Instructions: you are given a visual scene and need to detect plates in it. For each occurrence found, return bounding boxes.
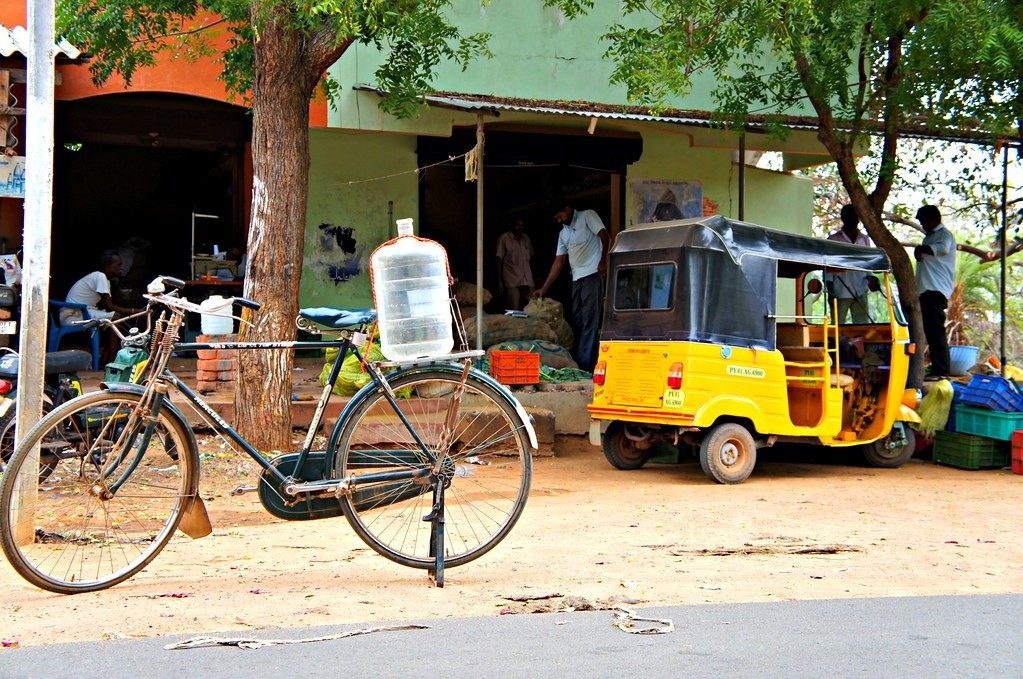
[197,253,209,257]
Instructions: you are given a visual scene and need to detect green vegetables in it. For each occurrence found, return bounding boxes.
[469,344,592,384]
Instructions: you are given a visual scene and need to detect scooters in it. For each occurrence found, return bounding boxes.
[0,287,191,485]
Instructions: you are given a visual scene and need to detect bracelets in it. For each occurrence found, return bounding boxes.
[132,308,134,314]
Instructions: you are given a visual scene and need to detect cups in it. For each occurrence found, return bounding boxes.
[214,245,219,255]
[218,253,223,261]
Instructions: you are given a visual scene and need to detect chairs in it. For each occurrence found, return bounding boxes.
[48,299,101,372]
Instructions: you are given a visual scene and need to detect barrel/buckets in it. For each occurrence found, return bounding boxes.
[201,295,233,335]
[948,345,978,376]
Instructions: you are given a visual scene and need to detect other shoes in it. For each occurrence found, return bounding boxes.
[98,359,122,374]
[925,371,949,380]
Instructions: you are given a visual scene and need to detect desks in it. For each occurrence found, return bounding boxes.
[164,279,243,342]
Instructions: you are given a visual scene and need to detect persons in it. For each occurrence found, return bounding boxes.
[914,205,956,382]
[824,203,870,324]
[533,199,610,373]
[496,219,535,310]
[59,249,150,367]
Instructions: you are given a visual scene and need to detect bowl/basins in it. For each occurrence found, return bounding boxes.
[200,275,206,281]
[211,276,218,282]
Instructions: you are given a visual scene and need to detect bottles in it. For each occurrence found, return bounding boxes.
[207,269,210,280]
[455,464,477,477]
[196,273,199,280]
[371,218,454,362]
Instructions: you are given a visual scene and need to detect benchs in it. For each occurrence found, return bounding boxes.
[775,321,854,387]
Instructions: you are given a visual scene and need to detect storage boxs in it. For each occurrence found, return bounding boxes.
[932,373,1023,475]
[489,349,540,384]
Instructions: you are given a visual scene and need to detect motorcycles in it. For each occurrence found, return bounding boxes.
[589,215,924,484]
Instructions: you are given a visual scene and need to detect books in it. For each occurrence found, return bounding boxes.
[512,314,527,317]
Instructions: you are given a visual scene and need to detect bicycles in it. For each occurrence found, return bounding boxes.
[0,276,539,594]
